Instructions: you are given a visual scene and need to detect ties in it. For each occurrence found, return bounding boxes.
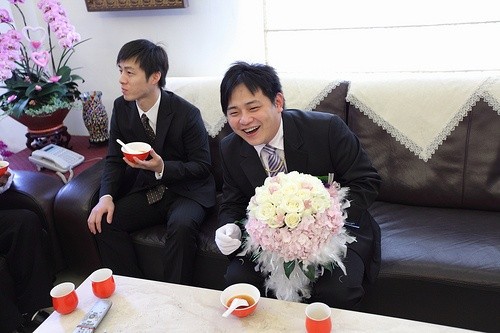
[260,144,287,177]
[139,114,166,205]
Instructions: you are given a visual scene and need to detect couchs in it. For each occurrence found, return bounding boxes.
[0,171,64,271]
[54,76,500,333]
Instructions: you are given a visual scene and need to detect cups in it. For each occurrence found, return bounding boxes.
[49,281,78,314]
[91,268,115,297]
[305,301,332,333]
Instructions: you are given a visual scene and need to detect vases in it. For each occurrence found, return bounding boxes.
[3,108,73,134]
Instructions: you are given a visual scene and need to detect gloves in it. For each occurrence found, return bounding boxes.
[216,223,242,255]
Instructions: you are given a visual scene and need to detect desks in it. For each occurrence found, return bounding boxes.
[32,269,483,333]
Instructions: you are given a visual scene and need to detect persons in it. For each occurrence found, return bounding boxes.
[0,156,51,333]
[216,61,384,312]
[86,39,216,286]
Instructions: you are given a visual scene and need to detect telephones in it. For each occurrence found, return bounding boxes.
[28,143,85,173]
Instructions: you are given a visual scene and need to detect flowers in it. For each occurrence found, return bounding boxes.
[243,171,350,279]
[0,0,92,118]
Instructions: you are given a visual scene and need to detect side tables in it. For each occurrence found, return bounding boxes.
[3,135,109,179]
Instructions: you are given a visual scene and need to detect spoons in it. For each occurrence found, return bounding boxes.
[222,297,248,317]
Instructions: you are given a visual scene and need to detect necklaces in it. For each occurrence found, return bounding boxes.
[262,150,286,173]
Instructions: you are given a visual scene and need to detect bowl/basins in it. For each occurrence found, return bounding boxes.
[0,161,9,176]
[220,283,260,317]
[121,141,151,162]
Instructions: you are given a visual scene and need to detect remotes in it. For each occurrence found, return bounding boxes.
[73,299,113,333]
